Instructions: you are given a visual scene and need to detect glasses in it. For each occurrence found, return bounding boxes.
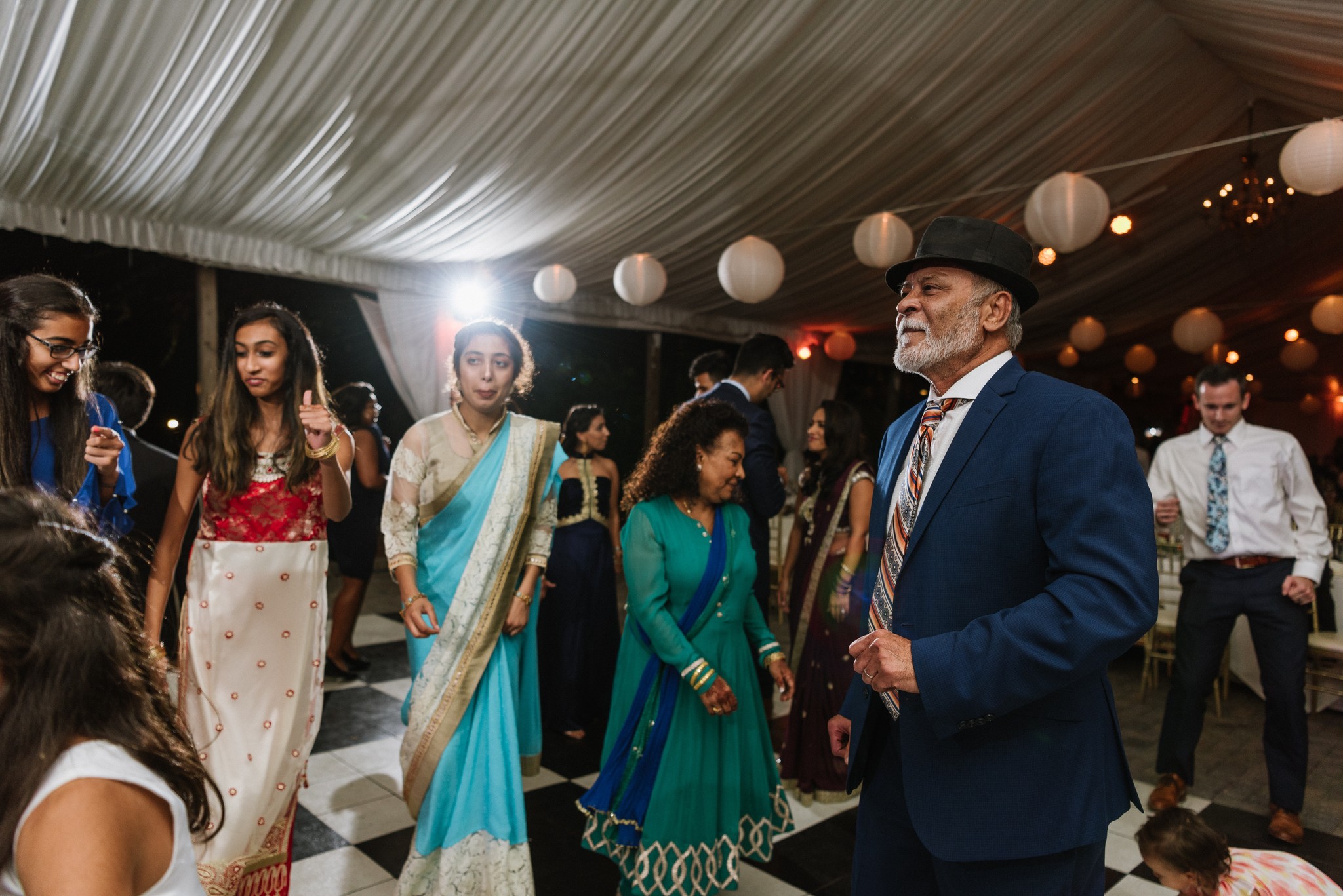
[24,331,101,361]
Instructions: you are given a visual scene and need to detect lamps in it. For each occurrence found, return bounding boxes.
[853,212,913,270]
[532,263,578,306]
[825,332,856,362]
[717,234,786,305]
[612,254,668,307]
[1025,114,1343,431]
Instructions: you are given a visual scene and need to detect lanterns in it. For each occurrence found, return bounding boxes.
[826,333,856,361]
[1056,286,1343,428]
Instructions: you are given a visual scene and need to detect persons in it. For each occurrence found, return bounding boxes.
[0,479,228,896]
[776,399,876,811]
[1133,806,1342,896]
[826,210,1162,896]
[379,319,568,896]
[323,380,392,681]
[150,304,355,896]
[573,394,795,896]
[1143,362,1332,844]
[687,329,796,748]
[537,403,626,751]
[87,359,202,670]
[0,272,138,548]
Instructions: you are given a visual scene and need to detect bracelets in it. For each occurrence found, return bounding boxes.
[514,591,533,606]
[145,642,168,667]
[304,432,342,462]
[757,641,785,668]
[399,592,427,620]
[680,658,715,691]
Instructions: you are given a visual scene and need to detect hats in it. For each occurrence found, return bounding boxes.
[882,211,1044,316]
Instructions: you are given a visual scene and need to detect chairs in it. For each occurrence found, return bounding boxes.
[1136,516,1343,729]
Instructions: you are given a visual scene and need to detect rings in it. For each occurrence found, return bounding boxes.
[864,667,874,679]
[713,707,722,714]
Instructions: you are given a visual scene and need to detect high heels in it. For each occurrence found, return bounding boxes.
[323,653,359,682]
[340,649,369,671]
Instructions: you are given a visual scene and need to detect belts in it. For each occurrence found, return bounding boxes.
[1213,549,1280,573]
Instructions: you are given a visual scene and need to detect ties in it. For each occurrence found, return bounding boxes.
[1204,433,1239,553]
[862,398,971,719]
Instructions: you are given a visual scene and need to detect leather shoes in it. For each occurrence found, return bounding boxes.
[1267,807,1305,846]
[1148,774,1187,814]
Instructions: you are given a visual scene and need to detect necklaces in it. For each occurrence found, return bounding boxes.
[681,496,715,523]
